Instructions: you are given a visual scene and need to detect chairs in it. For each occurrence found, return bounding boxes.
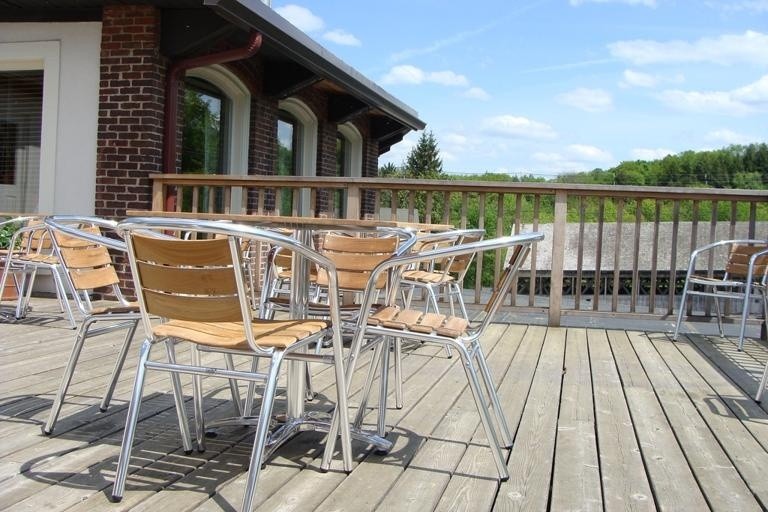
[673,239,767,353]
